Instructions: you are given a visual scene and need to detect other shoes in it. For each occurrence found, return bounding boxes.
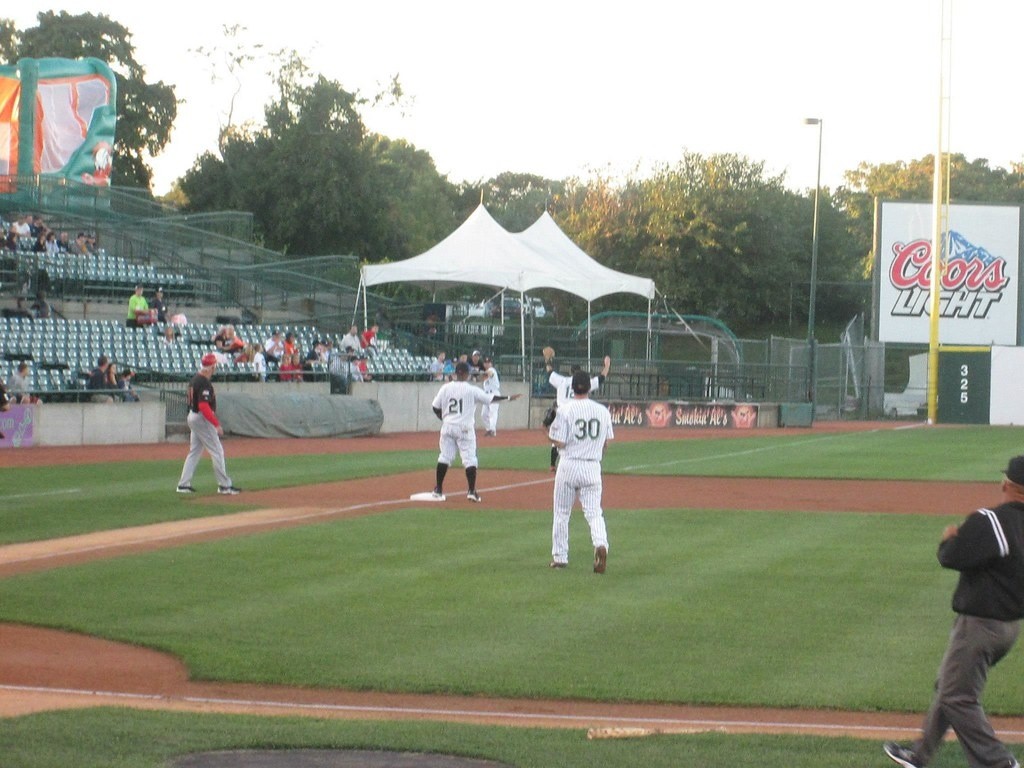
[484,430,494,437]
[593,546,606,574]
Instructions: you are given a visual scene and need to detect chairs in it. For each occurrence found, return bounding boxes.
[0,229,456,400]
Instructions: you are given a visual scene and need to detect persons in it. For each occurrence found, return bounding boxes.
[0,362,43,440]
[433,350,485,382]
[87,356,139,402]
[542,346,609,471]
[481,357,499,436]
[177,354,242,496]
[126,285,186,341]
[881,456,1024,768]
[213,324,379,383]
[433,363,522,503]
[547,371,614,574]
[0,213,101,256]
[543,401,557,473]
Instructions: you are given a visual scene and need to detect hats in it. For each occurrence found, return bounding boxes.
[472,350,480,357]
[351,356,359,361]
[202,354,218,366]
[483,357,492,363]
[1001,456,1024,485]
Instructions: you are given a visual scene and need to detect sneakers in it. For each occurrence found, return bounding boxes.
[176,485,195,492]
[432,491,442,498]
[883,741,923,768]
[549,562,567,567]
[1009,755,1020,768]
[217,486,240,495]
[467,490,481,502]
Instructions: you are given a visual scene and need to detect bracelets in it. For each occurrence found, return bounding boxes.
[508,395,510,401]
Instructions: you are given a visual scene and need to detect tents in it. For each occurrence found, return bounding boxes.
[361,189,591,376]
[506,206,658,376]
